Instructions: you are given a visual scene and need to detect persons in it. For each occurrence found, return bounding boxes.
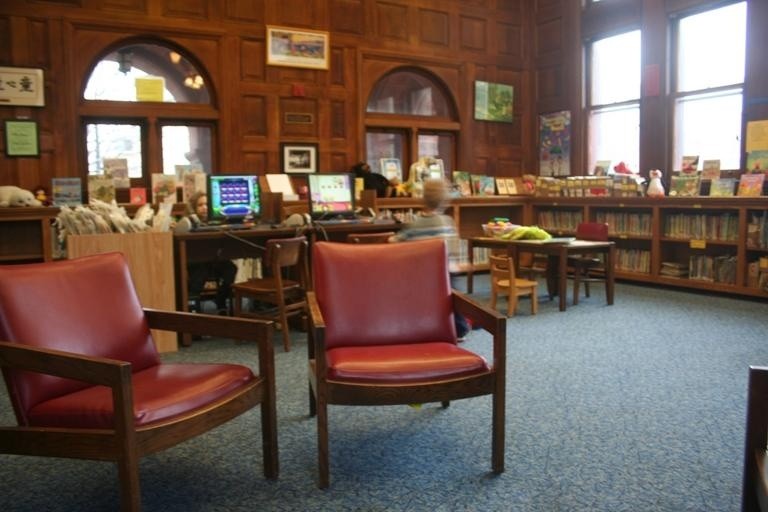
[187,191,236,341]
[387,179,470,342]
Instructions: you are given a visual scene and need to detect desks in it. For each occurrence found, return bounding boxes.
[466,237,621,311]
[172,218,409,348]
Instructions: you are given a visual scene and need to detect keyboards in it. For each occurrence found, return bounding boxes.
[191,225,250,232]
[315,220,360,226]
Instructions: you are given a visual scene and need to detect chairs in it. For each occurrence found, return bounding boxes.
[346,232,395,244]
[485,253,540,319]
[192,271,232,315]
[233,237,312,352]
[0,251,280,512]
[307,237,507,490]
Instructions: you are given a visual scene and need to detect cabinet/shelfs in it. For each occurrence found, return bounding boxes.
[376,197,767,301]
[0,207,60,262]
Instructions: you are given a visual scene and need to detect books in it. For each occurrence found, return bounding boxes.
[452,156,768,284]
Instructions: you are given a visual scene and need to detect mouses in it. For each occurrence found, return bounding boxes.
[396,218,403,223]
[271,222,279,228]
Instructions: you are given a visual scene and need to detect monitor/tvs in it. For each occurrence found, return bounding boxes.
[307,173,355,216]
[207,174,262,220]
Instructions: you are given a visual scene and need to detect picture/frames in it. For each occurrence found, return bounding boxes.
[280,142,319,174]
[266,24,330,70]
[380,158,402,183]
[536,108,572,177]
[5,119,40,155]
[0,67,44,107]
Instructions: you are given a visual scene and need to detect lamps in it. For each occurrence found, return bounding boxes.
[116,47,134,76]
